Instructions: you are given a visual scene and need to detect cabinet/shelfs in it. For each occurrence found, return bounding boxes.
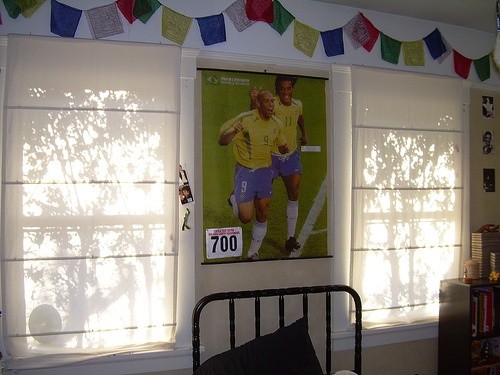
[437,277,500,375]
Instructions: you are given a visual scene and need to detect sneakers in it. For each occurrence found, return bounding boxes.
[284,236,301,250]
[228,190,235,208]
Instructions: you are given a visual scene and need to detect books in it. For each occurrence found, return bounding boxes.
[471,287,500,337]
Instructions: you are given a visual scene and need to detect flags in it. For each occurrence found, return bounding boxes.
[3,0,500,81]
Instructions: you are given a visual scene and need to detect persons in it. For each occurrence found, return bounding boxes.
[248,73,307,250]
[483,131,493,154]
[218,90,291,260]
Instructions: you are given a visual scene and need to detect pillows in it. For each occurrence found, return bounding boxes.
[196,317,324,375]
